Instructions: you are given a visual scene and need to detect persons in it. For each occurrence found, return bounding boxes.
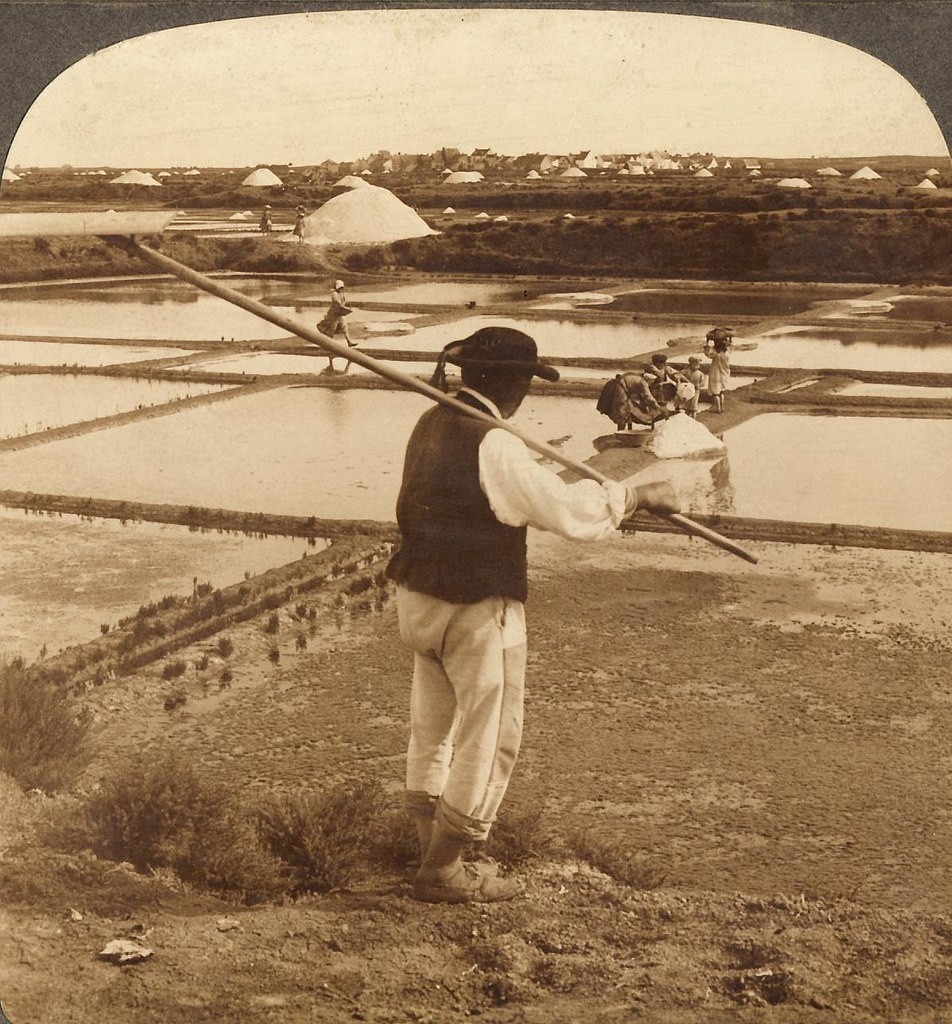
[597,332,734,433]
[322,356,353,376]
[259,205,272,237]
[317,278,359,346]
[293,204,306,242]
[388,326,682,902]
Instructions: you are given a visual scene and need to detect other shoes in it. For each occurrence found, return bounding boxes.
[411,857,526,903]
[348,343,358,347]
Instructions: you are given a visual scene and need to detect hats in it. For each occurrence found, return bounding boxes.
[651,354,668,363]
[688,356,703,362]
[333,279,344,290]
[429,327,560,393]
